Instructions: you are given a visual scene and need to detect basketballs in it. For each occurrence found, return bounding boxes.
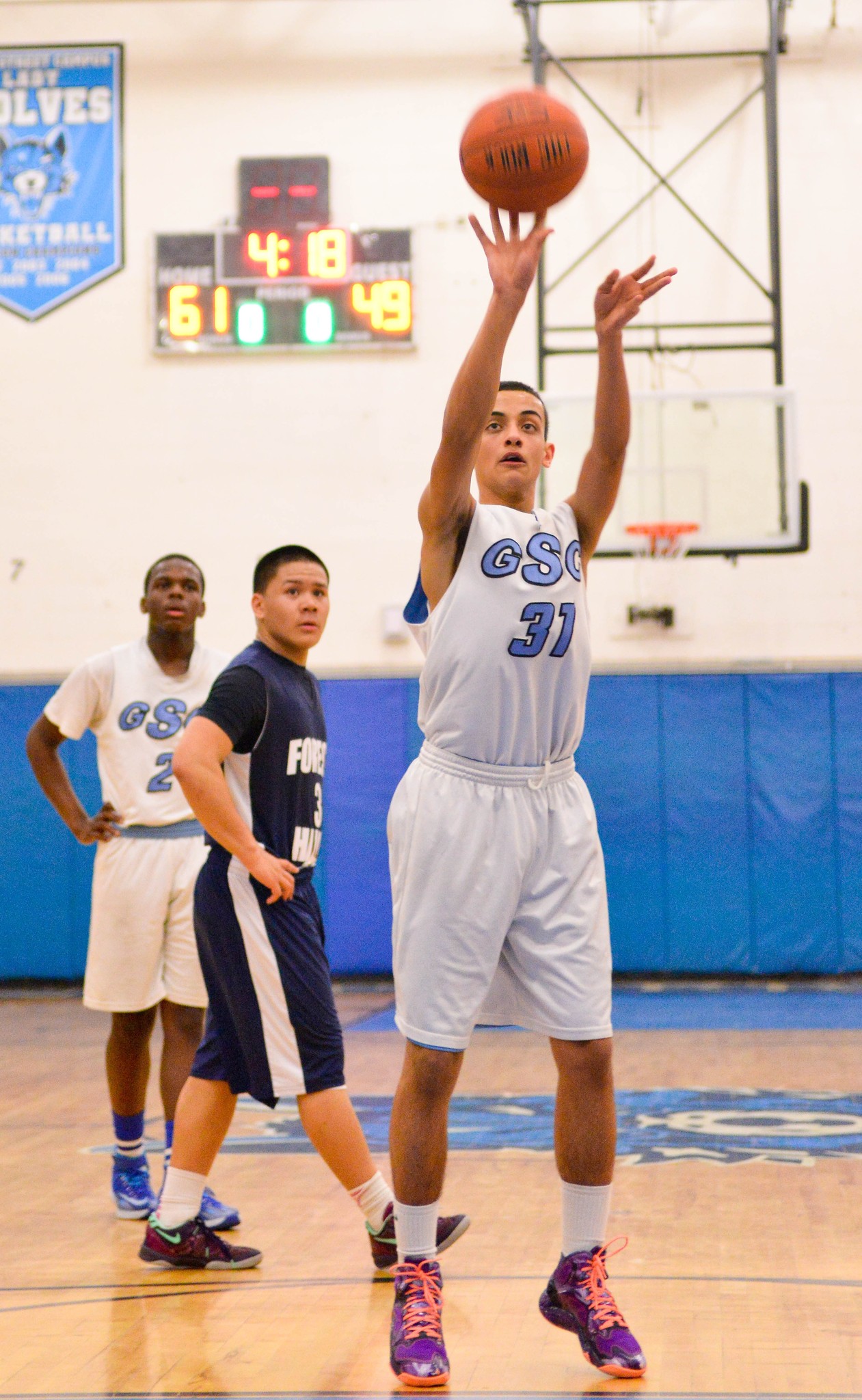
[459,89,591,213]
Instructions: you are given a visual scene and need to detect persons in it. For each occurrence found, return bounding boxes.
[141,545,472,1281]
[24,549,247,1233]
[386,196,683,1396]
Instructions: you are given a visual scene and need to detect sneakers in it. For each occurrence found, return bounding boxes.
[163,1175,239,1232]
[388,1256,451,1386]
[111,1162,159,1219]
[366,1200,471,1269]
[537,1237,646,1377]
[137,1213,261,1270]
